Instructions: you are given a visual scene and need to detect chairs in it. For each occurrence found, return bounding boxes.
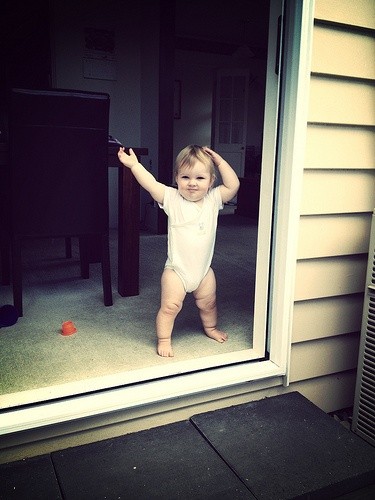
[2,83,114,319]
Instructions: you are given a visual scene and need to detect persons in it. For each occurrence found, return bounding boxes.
[119,144,242,357]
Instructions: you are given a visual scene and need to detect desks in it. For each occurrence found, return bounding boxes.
[105,134,149,300]
